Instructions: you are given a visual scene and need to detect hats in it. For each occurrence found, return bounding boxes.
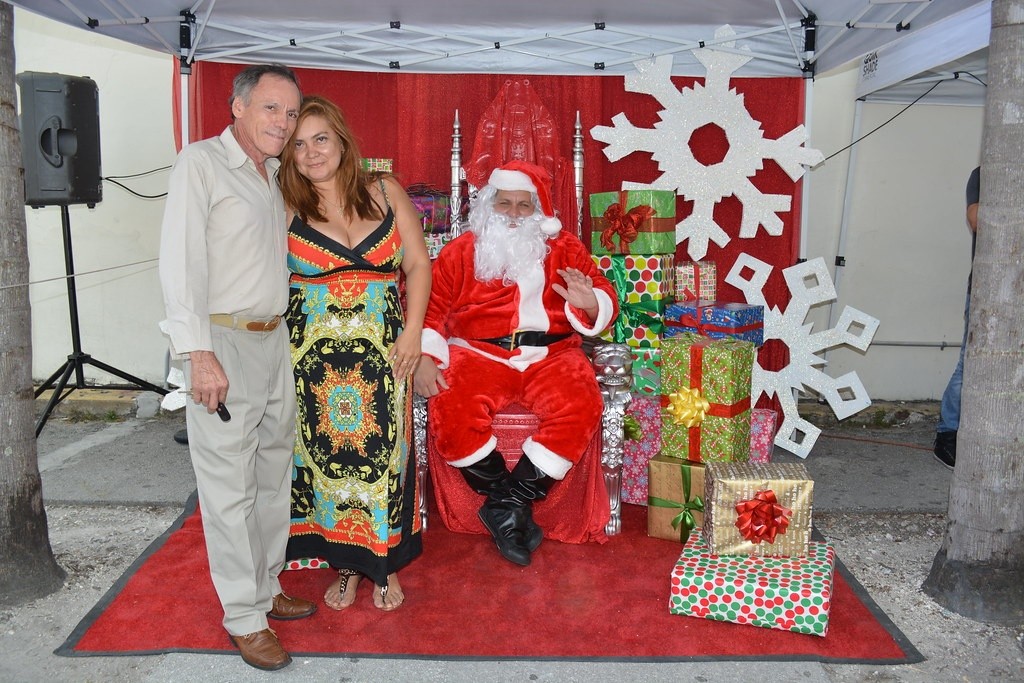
[487,161,561,236]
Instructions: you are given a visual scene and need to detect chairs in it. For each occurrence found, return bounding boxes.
[414,109,632,535]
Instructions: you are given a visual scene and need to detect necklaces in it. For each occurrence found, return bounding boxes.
[311,186,344,218]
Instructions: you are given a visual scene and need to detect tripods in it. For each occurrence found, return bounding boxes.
[34,203,171,442]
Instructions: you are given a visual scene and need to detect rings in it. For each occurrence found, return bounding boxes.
[418,392,424,395]
[402,360,408,365]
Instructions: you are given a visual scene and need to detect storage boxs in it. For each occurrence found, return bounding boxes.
[405,183,469,233]
[668,527,836,637]
[589,189,814,558]
[423,233,453,260]
[357,157,393,174]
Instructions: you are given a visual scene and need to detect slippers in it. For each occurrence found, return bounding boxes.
[372,572,400,611]
[324,571,359,609]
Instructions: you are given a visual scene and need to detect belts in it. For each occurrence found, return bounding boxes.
[476,329,572,353]
[209,312,282,331]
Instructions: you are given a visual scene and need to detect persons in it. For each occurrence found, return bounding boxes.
[932,165,981,463]
[157,63,317,670]
[276,96,432,610]
[414,160,619,568]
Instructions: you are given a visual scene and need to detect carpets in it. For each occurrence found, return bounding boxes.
[53,489,929,665]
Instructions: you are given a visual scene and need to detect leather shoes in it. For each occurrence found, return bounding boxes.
[266,593,317,621]
[229,629,292,671]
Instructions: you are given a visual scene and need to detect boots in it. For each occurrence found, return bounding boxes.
[476,451,554,566]
[459,447,544,553]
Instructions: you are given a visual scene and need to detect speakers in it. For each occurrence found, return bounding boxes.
[16,71,102,208]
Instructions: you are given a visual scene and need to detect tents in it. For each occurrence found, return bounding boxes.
[5,0,991,406]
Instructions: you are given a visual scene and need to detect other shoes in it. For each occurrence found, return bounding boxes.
[932,435,957,468]
